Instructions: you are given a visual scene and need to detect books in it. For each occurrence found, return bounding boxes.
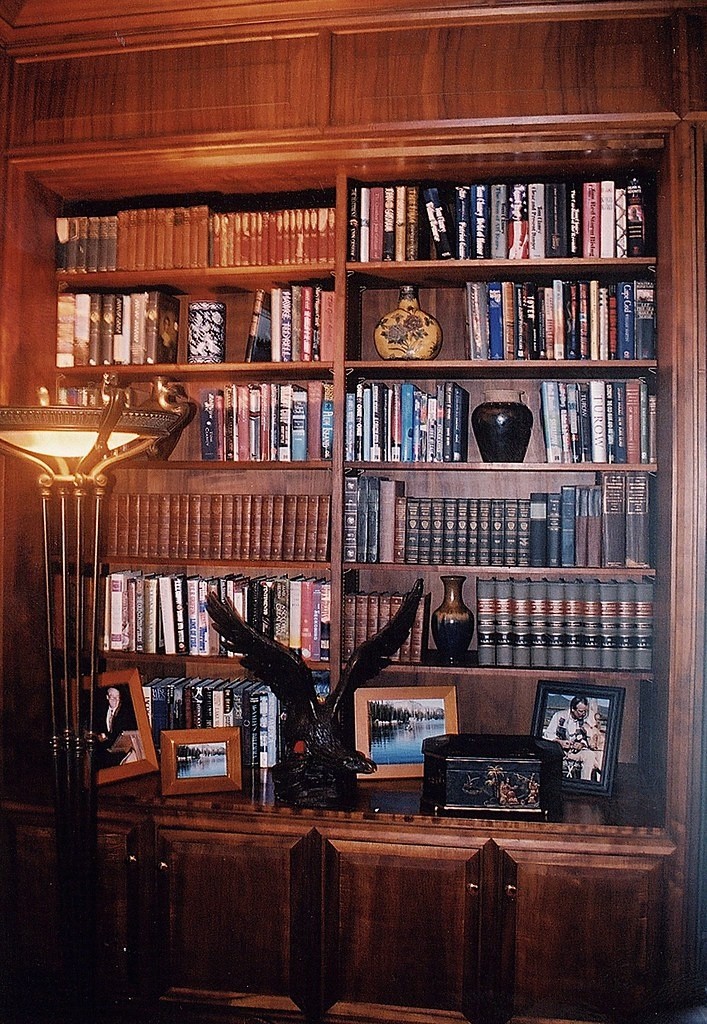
[199,380,337,464]
[343,470,651,569]
[102,491,334,562]
[343,377,469,462]
[347,176,656,261]
[476,576,653,671]
[53,206,339,274]
[137,678,325,805]
[539,378,658,465]
[107,572,330,660]
[465,276,658,362]
[344,592,431,665]
[55,286,333,366]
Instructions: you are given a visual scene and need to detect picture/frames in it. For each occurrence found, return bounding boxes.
[158,724,243,797]
[532,680,627,798]
[59,667,160,787]
[420,731,563,822]
[353,686,457,780]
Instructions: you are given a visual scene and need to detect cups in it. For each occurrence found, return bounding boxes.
[187,301,225,364]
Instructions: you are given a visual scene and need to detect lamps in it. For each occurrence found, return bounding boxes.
[0,373,199,1024]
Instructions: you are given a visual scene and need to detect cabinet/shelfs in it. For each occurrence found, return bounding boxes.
[0,106,707,1023]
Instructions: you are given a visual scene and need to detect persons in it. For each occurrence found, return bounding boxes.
[546,695,604,778]
[94,689,128,768]
